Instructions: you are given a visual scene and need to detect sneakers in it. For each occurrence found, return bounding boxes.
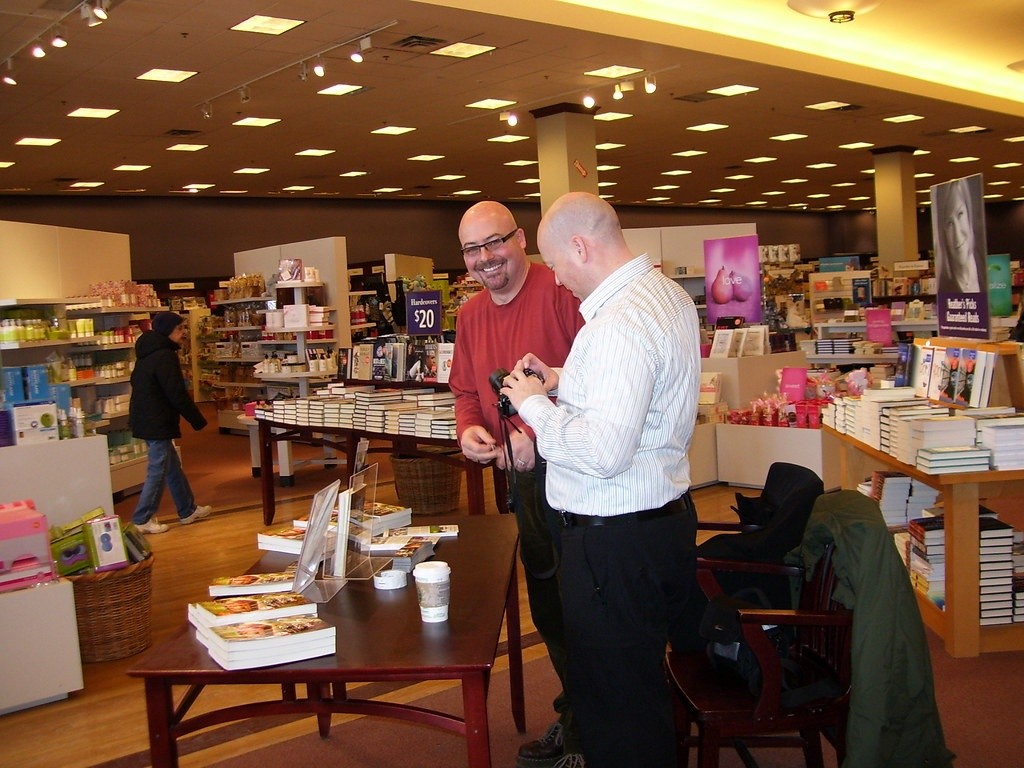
[133,520,168,534]
[179,505,212,523]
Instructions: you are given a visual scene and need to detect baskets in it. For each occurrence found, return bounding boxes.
[58,552,156,663]
[390,452,462,515]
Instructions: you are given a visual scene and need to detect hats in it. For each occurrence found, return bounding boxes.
[152,312,184,336]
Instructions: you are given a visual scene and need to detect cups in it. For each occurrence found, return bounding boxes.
[412,561,451,622]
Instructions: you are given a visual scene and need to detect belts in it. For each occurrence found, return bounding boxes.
[549,492,693,527]
[526,464,546,476]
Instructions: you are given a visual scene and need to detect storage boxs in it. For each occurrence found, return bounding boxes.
[48,506,152,577]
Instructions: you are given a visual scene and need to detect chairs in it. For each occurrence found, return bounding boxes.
[665,520,854,768]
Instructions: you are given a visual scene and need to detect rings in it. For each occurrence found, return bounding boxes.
[518,459,525,466]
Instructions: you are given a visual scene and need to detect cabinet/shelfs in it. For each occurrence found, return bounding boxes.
[348,254,486,341]
[208,282,342,434]
[660,261,1024,659]
[0,298,181,496]
[177,308,212,401]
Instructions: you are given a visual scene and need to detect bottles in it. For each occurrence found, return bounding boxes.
[71,329,130,378]
[57,407,86,440]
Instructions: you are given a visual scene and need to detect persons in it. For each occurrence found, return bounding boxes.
[936,178,986,294]
[447,201,586,768]
[500,192,702,768]
[125,312,212,532]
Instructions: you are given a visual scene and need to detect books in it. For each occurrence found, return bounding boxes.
[253,382,457,439]
[823,386,1024,475]
[187,572,336,672]
[800,337,863,354]
[857,471,1024,626]
[881,346,898,354]
[337,342,455,383]
[257,501,413,555]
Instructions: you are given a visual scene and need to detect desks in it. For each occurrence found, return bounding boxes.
[238,411,509,525]
[125,516,526,768]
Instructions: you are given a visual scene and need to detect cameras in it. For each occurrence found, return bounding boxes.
[489,368,544,418]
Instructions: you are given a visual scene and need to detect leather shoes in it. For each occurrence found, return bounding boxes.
[515,721,573,768]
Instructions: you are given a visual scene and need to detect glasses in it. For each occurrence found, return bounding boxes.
[460,229,518,258]
